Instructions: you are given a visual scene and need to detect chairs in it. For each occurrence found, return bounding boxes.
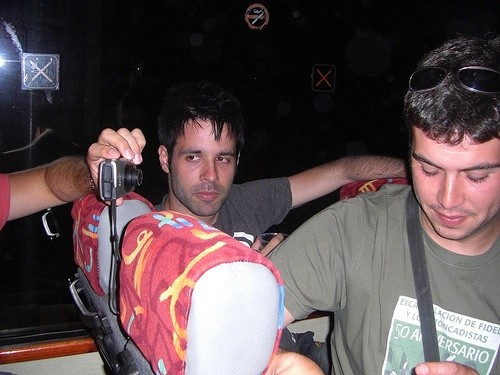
[70,191,285,375]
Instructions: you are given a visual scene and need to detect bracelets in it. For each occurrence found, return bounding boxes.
[88,175,95,192]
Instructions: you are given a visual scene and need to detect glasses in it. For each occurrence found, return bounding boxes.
[408,66,500,96]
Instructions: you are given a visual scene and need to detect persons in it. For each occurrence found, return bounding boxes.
[0,128,145,228]
[154,87,406,375]
[264,37,500,375]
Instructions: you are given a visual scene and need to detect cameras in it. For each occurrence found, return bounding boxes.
[99,157,143,202]
